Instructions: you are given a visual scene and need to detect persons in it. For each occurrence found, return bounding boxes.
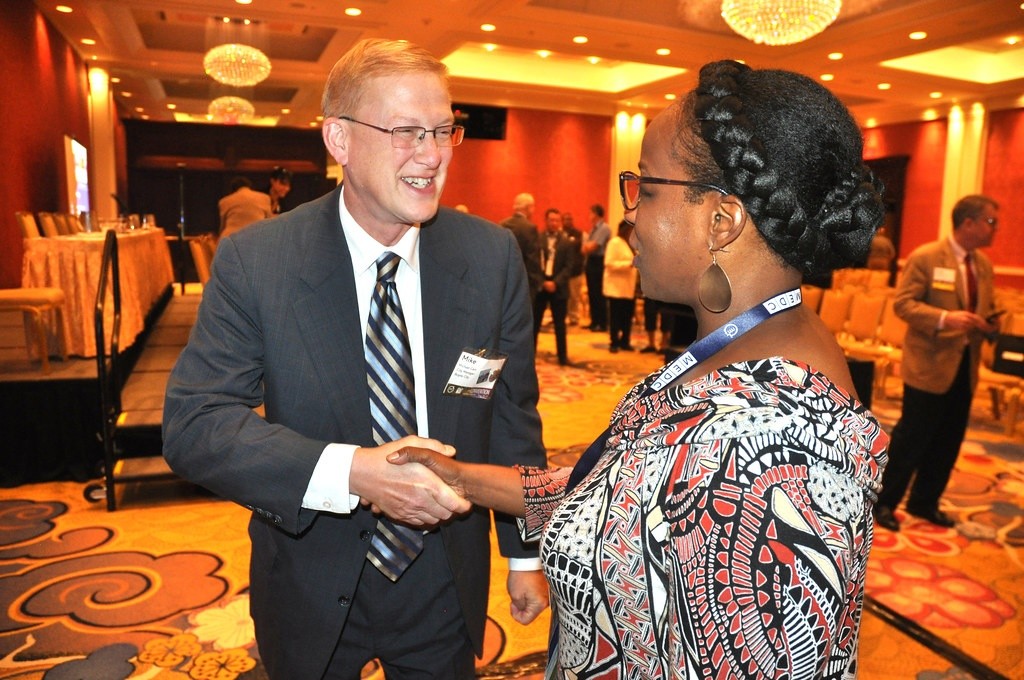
[531,208,577,364]
[219,178,271,236]
[876,195,1005,529]
[847,236,896,407]
[563,212,589,326]
[161,38,551,680]
[603,219,640,352]
[581,204,612,332]
[359,59,893,679]
[268,166,293,215]
[642,297,674,354]
[501,193,542,304]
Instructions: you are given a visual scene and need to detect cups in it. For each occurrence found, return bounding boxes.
[128,214,140,231]
[142,214,156,230]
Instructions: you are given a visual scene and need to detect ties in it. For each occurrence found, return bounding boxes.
[589,225,597,239]
[964,254,978,342]
[366,251,424,582]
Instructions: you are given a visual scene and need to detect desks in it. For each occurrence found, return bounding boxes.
[19,226,175,360]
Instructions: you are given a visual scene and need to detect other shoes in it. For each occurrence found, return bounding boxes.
[582,322,608,332]
[640,345,657,354]
[876,510,900,532]
[927,510,956,528]
[621,346,635,351]
[609,346,618,353]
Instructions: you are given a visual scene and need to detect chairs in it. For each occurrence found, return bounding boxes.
[794,267,1024,429]
[0,203,218,374]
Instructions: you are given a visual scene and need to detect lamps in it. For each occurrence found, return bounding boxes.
[207,96,256,124]
[721,0,842,48]
[204,43,272,86]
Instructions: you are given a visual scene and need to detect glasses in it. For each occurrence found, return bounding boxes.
[339,116,465,149]
[978,215,998,227]
[619,170,729,211]
